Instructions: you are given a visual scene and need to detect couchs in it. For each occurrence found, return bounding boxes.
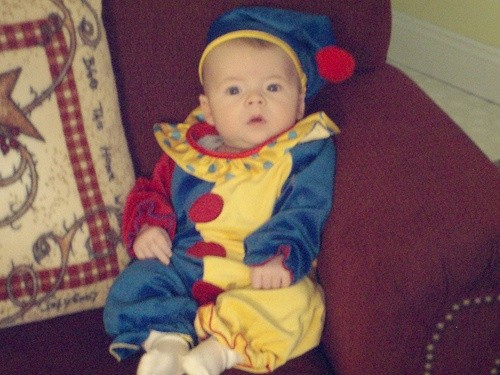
[1,1,500,375]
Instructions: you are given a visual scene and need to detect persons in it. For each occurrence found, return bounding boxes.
[102,5,343,375]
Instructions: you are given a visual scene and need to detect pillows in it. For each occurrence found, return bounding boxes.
[0,0,137,330]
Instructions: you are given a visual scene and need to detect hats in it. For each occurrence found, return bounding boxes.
[198,9,356,97]
